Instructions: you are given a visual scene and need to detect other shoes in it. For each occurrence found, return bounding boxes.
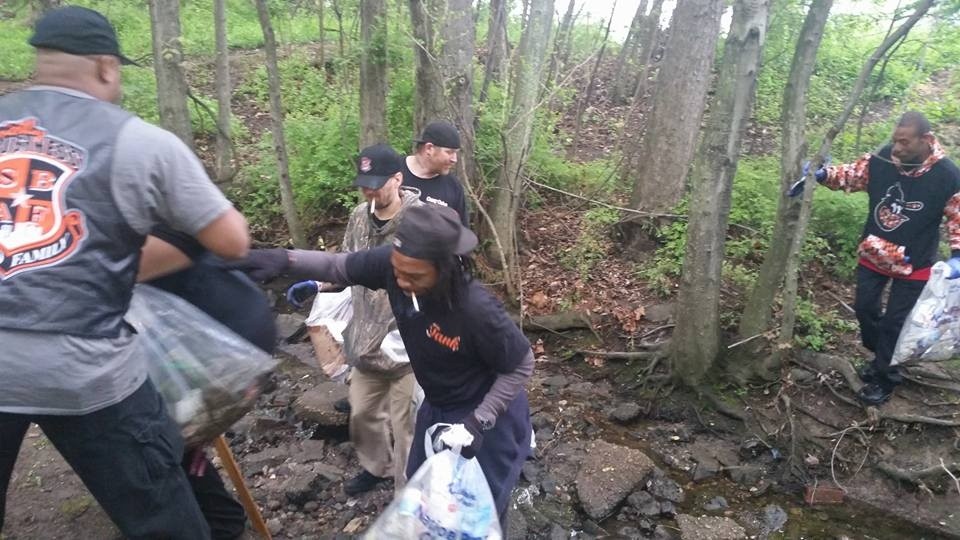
[344,471,385,492]
[334,397,352,413]
[857,380,895,404]
[860,360,884,383]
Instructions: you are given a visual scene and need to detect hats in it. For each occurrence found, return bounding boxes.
[357,143,401,190]
[392,201,478,259]
[413,121,460,149]
[27,5,142,67]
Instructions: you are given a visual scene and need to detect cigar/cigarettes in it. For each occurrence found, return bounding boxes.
[410,290,420,312]
[370,197,376,214]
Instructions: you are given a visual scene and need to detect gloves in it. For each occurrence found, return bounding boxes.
[443,413,485,459]
[225,247,288,285]
[803,160,825,182]
[287,280,318,312]
[945,257,960,279]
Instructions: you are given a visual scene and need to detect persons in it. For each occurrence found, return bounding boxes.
[204,203,537,540]
[399,123,468,229]
[1,6,279,540]
[802,111,960,406]
[286,142,425,495]
